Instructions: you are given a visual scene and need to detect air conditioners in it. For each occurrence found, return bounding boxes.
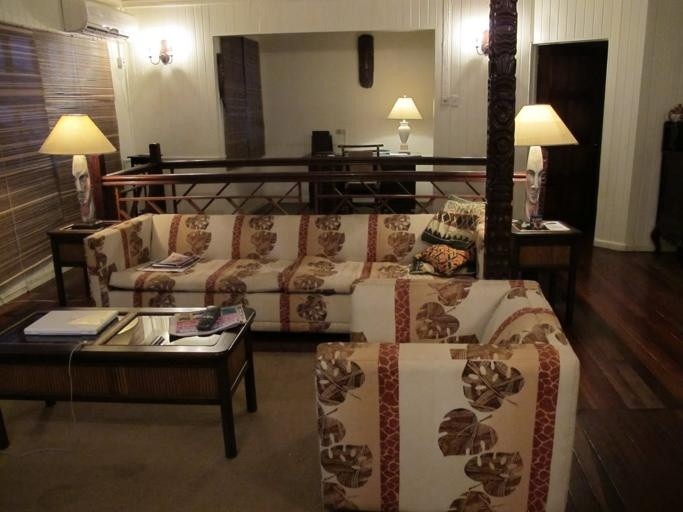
[61,0,135,42]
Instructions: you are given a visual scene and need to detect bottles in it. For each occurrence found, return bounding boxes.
[335,128,345,154]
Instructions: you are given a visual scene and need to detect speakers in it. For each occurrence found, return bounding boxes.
[358,34,375,89]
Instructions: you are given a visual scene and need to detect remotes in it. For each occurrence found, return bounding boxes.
[196,304,221,330]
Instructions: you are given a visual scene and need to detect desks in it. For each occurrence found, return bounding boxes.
[47,219,122,307]
[512,221,584,328]
[299,154,420,209]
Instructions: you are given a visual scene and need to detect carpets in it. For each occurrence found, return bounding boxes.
[0,350,322,512]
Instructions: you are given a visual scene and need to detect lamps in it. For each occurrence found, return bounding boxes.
[515,103,579,232]
[387,97,424,155]
[31,114,117,234]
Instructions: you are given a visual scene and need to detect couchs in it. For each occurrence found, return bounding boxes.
[83,213,484,333]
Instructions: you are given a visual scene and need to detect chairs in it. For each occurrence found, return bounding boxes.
[331,144,397,214]
[315,276,582,511]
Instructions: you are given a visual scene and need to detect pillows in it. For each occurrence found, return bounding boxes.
[410,193,487,277]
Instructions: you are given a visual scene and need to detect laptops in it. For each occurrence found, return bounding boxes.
[24,309,119,336]
